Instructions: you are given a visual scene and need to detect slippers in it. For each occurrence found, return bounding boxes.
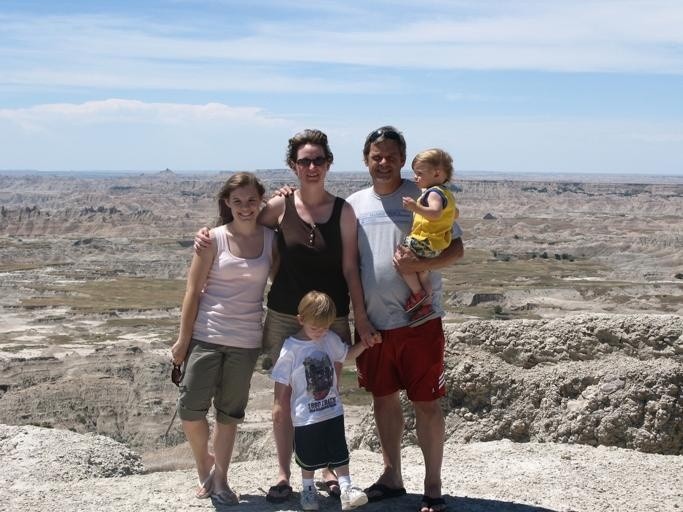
[195,467,444,511]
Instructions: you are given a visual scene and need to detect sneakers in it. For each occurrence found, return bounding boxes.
[408,305,439,328]
[405,289,431,313]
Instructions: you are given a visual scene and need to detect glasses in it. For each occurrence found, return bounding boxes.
[369,130,399,142]
[297,157,327,166]
[171,362,181,386]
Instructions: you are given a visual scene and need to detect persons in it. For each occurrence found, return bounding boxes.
[271,124,465,512]
[390,148,458,324]
[272,289,383,512]
[252,129,382,504]
[170,171,282,508]
